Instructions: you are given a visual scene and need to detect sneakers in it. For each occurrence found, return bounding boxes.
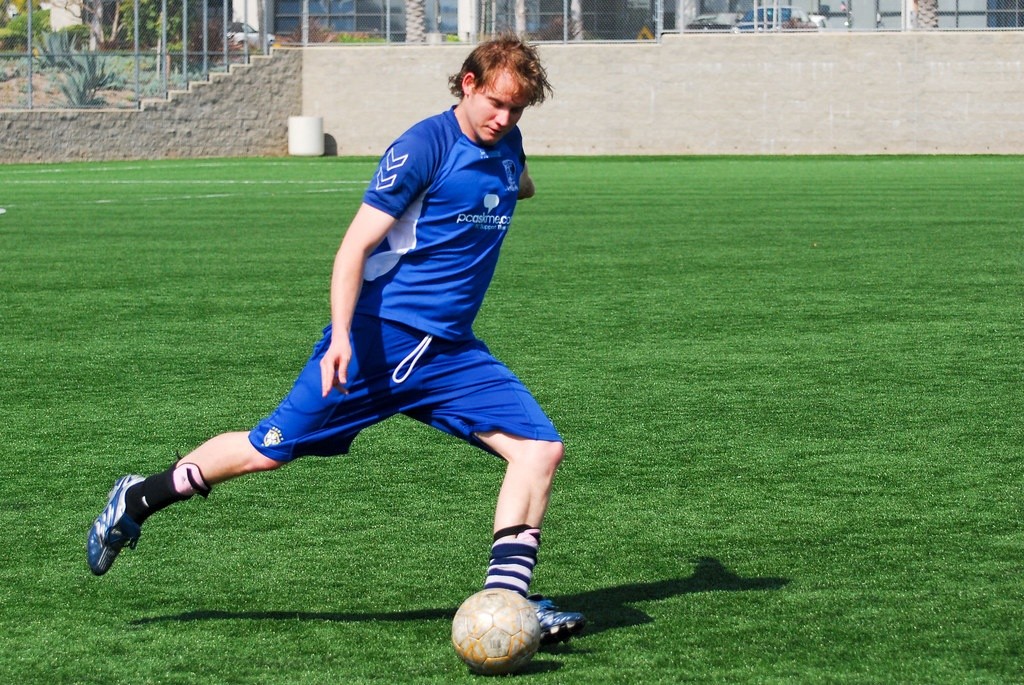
[526,594,584,645]
[87,474,146,576]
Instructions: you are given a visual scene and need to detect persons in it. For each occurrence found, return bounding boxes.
[85,37,589,647]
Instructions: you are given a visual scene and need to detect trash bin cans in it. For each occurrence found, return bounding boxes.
[287,115,325,157]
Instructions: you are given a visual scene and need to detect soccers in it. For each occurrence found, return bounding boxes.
[450,588,542,676]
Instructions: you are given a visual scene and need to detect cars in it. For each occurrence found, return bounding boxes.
[685,13,743,33]
[732,6,818,32]
